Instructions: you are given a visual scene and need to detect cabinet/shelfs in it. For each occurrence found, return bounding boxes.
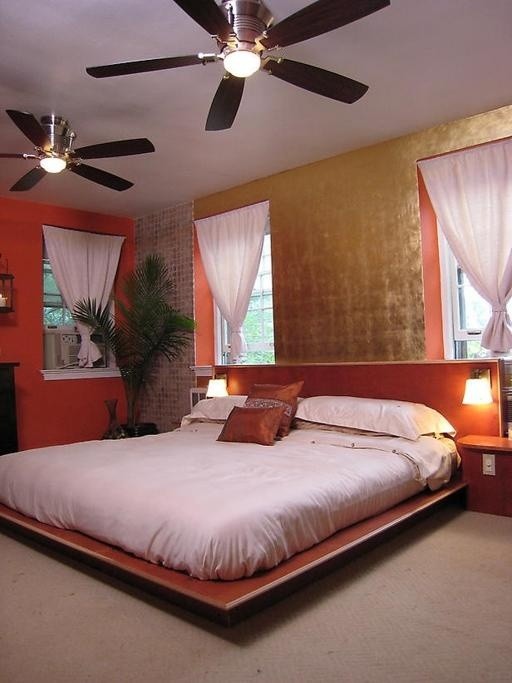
[0,361,22,457]
[1,274,15,313]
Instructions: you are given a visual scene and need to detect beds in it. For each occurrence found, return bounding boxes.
[0,422,469,628]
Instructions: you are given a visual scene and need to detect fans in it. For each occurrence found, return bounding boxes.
[85,1,391,132]
[0,110,155,192]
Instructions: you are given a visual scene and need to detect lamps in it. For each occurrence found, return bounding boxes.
[206,374,228,397]
[39,158,66,173]
[462,366,492,406]
[223,51,261,78]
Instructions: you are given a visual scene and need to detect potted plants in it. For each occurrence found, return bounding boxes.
[71,252,196,438]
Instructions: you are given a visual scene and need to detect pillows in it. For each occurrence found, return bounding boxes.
[181,396,248,426]
[296,396,457,440]
[243,381,304,441]
[216,406,288,445]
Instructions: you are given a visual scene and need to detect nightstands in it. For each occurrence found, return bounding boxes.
[458,435,512,517]
